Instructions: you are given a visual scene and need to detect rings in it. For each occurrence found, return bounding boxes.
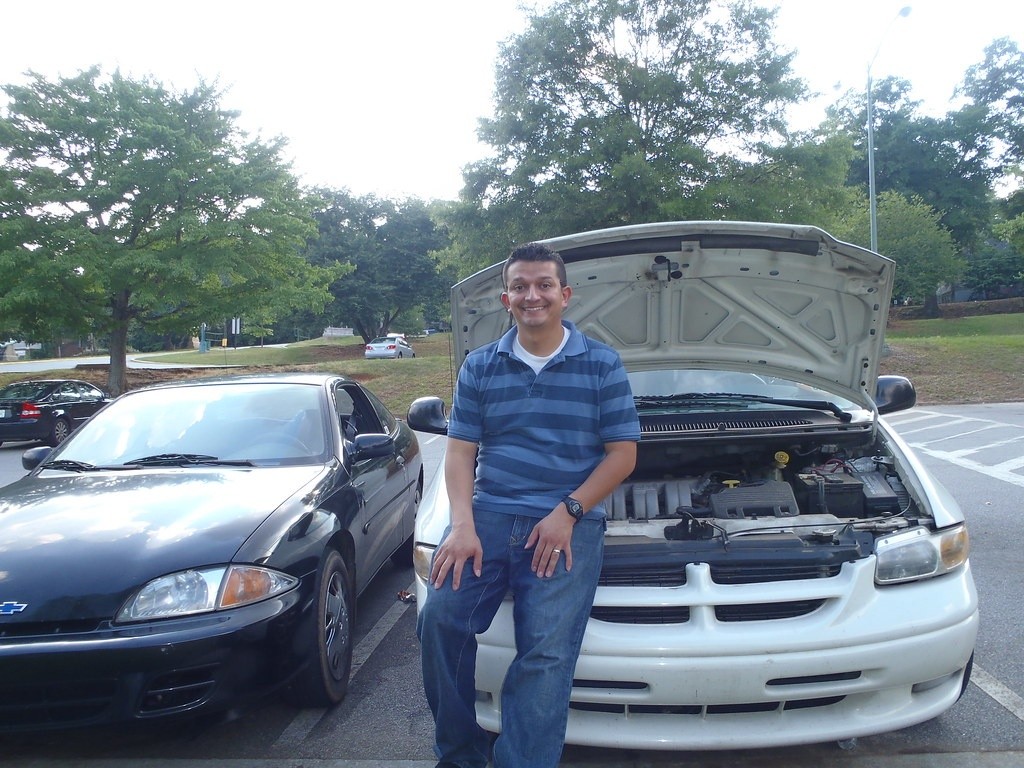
[553,550,560,553]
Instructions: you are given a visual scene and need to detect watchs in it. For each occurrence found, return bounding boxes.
[561,496,583,521]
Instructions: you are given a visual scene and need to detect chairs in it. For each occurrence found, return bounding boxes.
[283,409,354,452]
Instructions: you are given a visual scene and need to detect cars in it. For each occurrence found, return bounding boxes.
[0,379,113,448]
[0,372,424,741]
[365,337,416,359]
[405,220,981,752]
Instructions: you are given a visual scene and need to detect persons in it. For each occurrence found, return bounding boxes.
[415,243,641,767]
[905,296,912,306]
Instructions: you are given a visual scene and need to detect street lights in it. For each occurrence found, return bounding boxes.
[866,7,914,253]
